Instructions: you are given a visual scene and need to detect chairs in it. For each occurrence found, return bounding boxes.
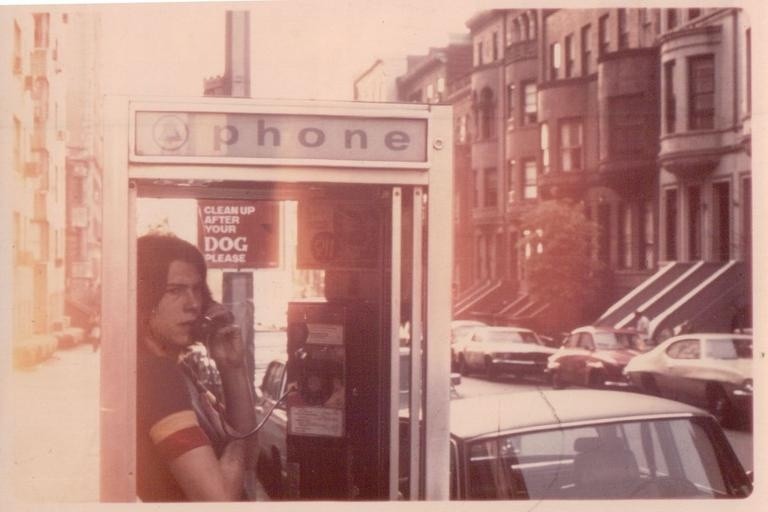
[453,454,525,499]
[559,449,659,499]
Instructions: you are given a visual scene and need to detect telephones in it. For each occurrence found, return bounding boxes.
[195,282,214,342]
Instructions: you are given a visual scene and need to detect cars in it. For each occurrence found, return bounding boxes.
[543,323,648,390]
[622,331,752,426]
[450,319,493,372]
[393,347,753,503]
[454,326,562,382]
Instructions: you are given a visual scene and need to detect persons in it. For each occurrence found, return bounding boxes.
[726,303,745,334]
[626,309,651,346]
[136,232,266,503]
